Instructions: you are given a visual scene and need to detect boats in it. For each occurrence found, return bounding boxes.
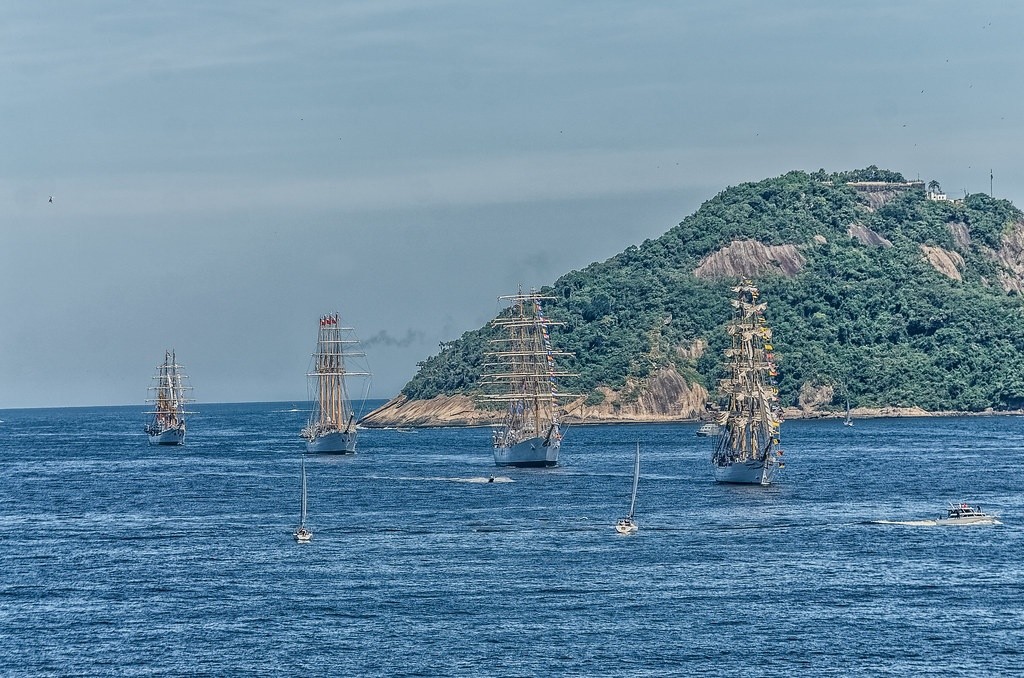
[696,422,721,436]
[935,503,1003,526]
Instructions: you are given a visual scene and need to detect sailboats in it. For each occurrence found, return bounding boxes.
[615,442,639,533]
[843,399,853,427]
[478,284,585,469]
[141,349,200,446]
[304,312,374,455]
[710,277,786,486]
[292,454,313,541]
